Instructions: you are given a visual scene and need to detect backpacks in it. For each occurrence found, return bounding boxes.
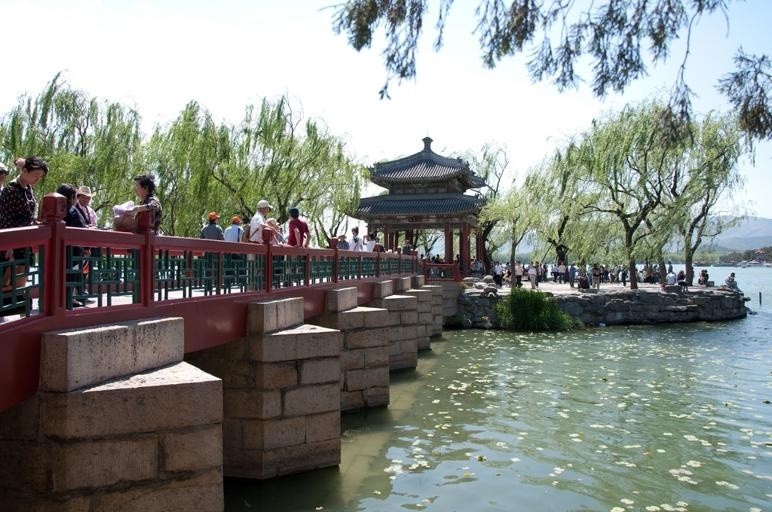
[241,222,260,242]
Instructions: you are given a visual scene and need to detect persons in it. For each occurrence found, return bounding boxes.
[201,201,312,295]
[347,225,363,251]
[113,175,163,304]
[336,234,350,280]
[0,156,100,319]
[419,254,487,277]
[724,272,743,292]
[363,232,376,252]
[372,238,418,255]
[491,260,709,291]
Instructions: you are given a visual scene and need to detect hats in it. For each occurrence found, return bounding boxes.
[288,208,298,212]
[257,199,273,209]
[77,185,94,197]
[208,211,220,219]
[266,218,279,225]
[232,216,240,223]
